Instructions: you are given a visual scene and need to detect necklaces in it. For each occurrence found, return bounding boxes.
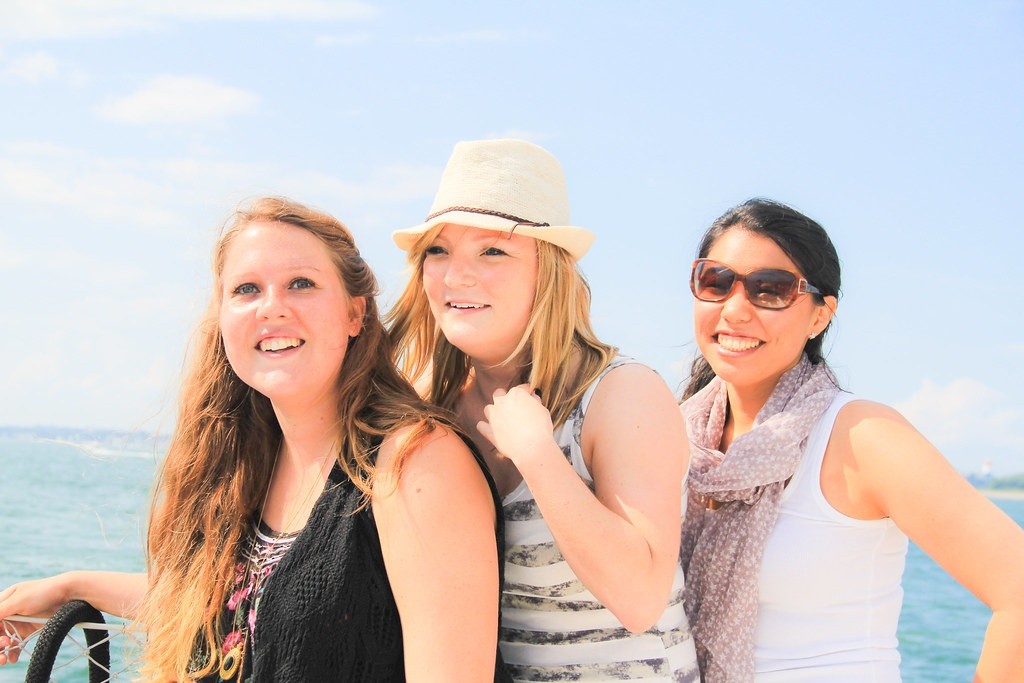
[220,435,335,680]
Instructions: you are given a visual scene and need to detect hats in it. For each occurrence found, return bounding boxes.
[392,138,595,263]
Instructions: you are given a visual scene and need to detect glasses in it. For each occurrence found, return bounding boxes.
[690,258,819,310]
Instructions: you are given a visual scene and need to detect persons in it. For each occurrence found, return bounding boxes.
[681,198,1024,683]
[380,140,700,683]
[0,200,505,683]
